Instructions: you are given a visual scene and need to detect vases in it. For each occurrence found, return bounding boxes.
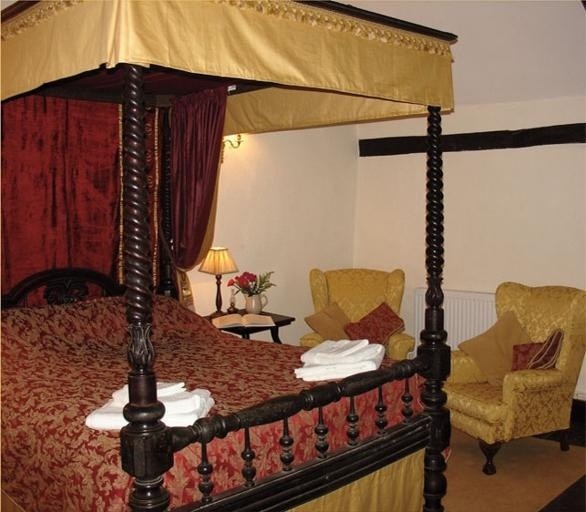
[244,295,268,314]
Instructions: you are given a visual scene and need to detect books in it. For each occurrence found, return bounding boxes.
[211,312,276,329]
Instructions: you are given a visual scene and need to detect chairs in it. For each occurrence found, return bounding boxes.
[443,281,586,476]
[301,265,415,360]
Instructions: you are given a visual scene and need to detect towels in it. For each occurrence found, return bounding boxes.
[85,376,215,429]
[293,339,386,383]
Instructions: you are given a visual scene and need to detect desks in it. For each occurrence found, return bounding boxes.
[202,308,296,345]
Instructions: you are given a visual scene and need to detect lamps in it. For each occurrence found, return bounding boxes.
[221,133,244,162]
[196,246,238,318]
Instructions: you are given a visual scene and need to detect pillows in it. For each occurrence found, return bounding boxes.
[513,340,545,372]
[344,301,407,347]
[459,310,535,389]
[528,329,564,370]
[303,302,349,341]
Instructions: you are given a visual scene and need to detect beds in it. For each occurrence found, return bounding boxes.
[1,0,459,511]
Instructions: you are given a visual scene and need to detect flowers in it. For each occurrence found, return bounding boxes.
[226,270,275,293]
